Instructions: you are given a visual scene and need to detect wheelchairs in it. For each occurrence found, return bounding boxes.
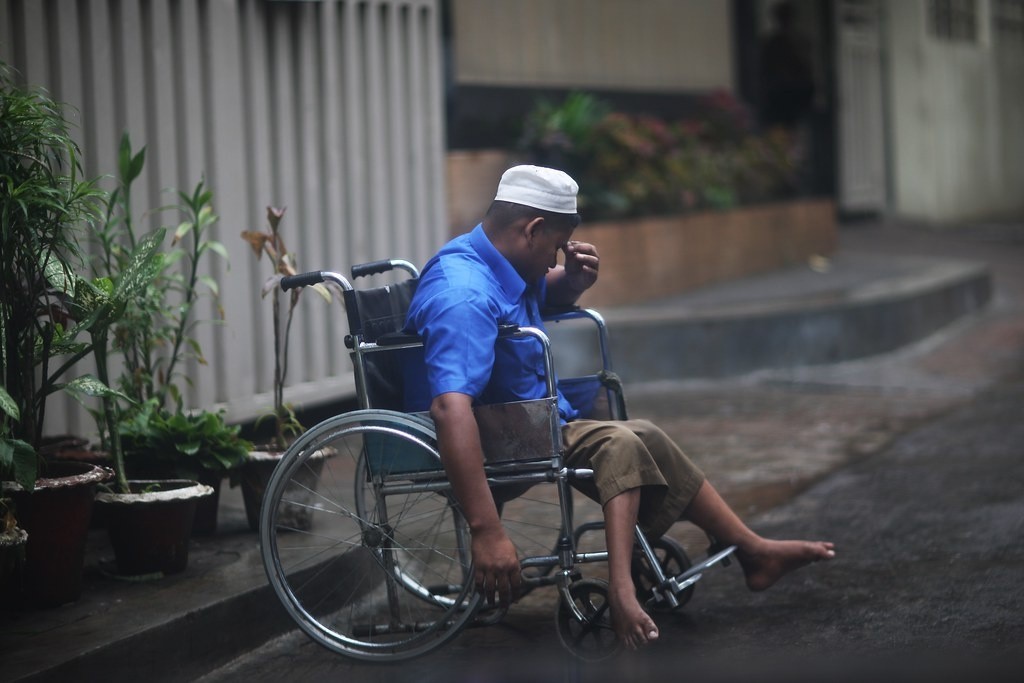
[257,258,759,666]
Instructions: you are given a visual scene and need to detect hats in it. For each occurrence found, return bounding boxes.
[493,164,580,214]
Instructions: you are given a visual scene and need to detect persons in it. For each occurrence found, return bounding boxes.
[398,165,837,654]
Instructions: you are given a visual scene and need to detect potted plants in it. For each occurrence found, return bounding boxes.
[0,58,342,583]
